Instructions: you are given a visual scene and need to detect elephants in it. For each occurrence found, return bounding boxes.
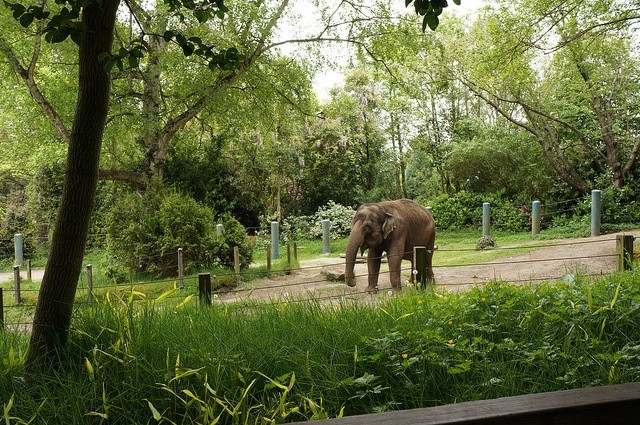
[345,198,436,295]
